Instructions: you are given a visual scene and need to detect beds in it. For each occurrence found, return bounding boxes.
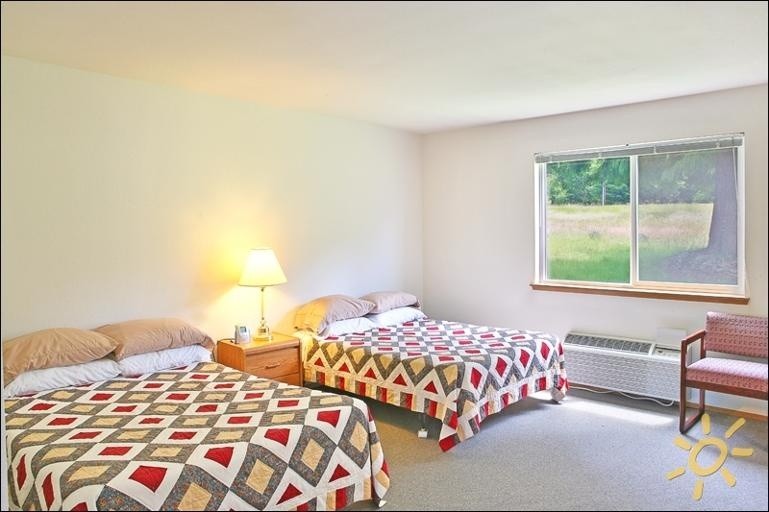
[272,319,563,442]
[3,362,370,511]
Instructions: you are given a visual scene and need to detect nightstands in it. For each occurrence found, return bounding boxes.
[217,332,303,387]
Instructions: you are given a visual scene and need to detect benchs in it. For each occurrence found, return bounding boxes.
[680,311,768,435]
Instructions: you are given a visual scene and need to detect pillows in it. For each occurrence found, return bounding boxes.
[285,292,429,342]
[3,318,218,400]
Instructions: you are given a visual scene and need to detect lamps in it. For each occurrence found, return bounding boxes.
[238,248,288,341]
